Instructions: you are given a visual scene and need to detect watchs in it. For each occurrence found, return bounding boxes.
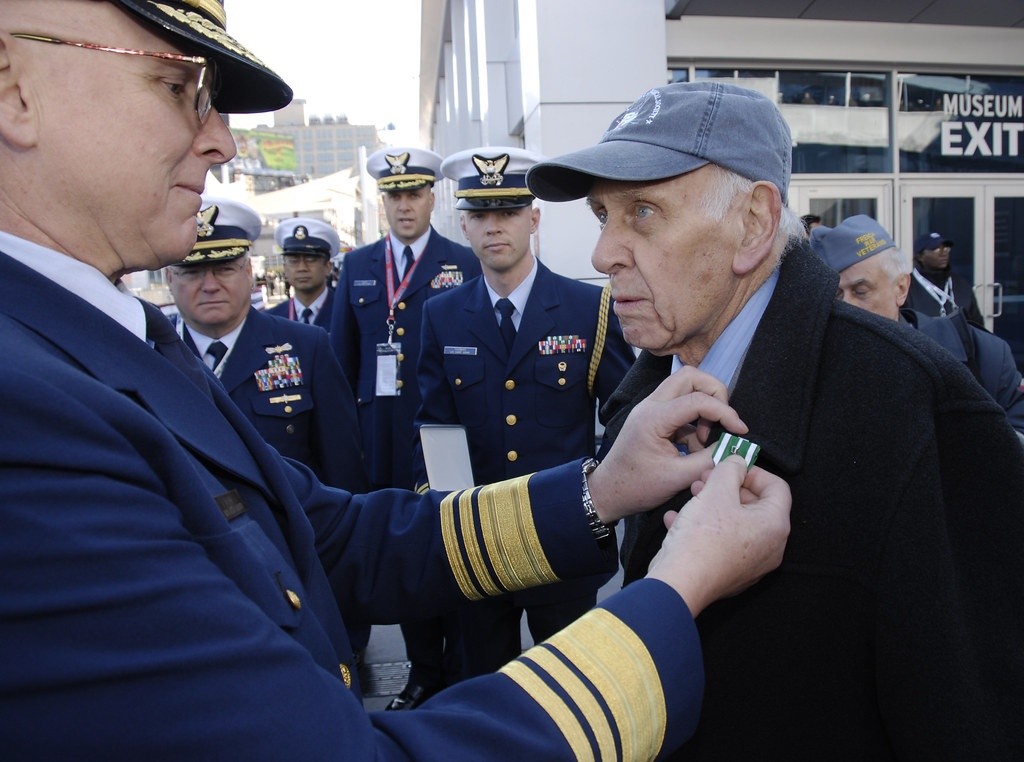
[580,457,611,540]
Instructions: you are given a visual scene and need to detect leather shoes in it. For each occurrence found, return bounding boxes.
[384,667,441,713]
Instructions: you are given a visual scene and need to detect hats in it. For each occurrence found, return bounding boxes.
[166,196,262,265]
[524,81,792,204]
[368,146,444,191]
[122,0,293,115]
[809,213,897,274]
[912,233,953,253]
[275,217,340,265]
[441,147,549,209]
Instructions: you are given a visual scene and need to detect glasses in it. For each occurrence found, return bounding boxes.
[933,246,950,252]
[10,31,218,124]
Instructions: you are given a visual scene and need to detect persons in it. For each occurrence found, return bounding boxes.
[330,146,484,496]
[0,0,793,761]
[808,213,1024,438]
[150,197,374,676]
[905,230,993,336]
[525,80,1024,762]
[798,214,821,238]
[384,147,638,713]
[262,217,338,333]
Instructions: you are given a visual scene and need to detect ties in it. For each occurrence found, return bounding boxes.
[405,245,415,280]
[496,298,518,350]
[303,309,314,325]
[208,340,229,372]
